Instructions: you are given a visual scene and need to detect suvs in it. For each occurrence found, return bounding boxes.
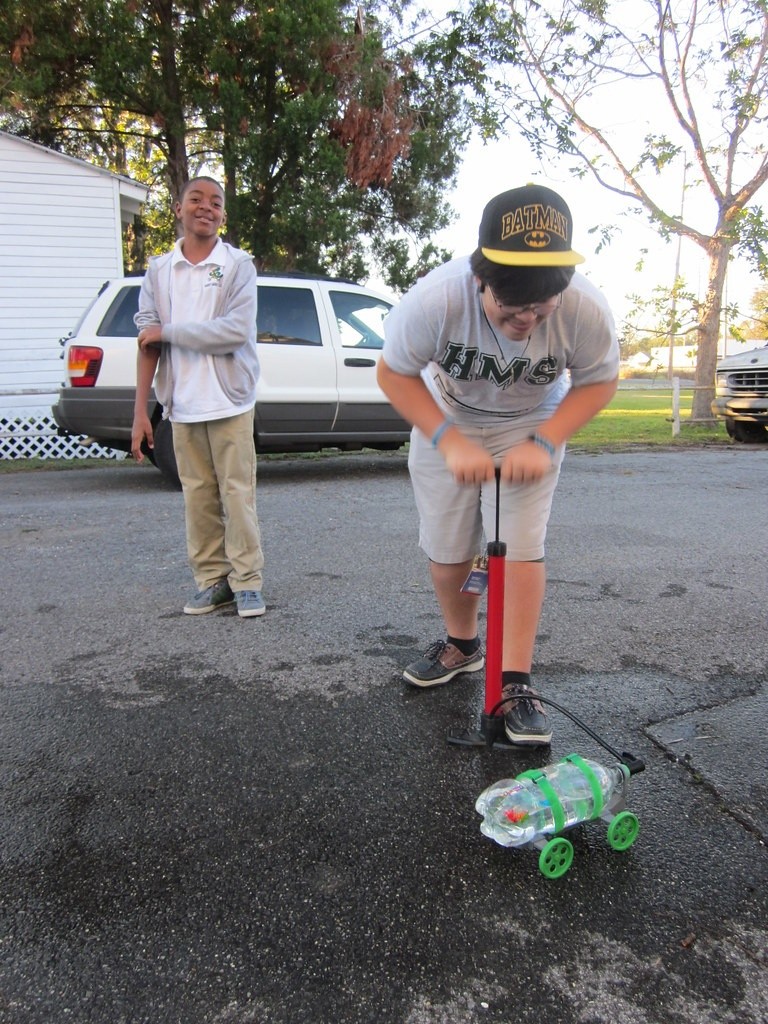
[712,342,768,444]
[52,270,415,491]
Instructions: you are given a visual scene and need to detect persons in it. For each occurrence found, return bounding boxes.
[376,181,623,746]
[130,176,271,619]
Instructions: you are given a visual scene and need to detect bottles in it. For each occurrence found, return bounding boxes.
[474,756,631,848]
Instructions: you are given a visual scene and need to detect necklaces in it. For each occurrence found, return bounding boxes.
[481,308,531,369]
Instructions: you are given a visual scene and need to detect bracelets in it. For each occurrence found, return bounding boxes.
[431,419,452,449]
[526,434,556,456]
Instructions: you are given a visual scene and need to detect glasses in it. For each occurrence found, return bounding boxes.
[485,281,563,316]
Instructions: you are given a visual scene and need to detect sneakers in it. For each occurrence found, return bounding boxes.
[402,639,485,688]
[183,579,236,614]
[233,591,266,617]
[499,682,554,745]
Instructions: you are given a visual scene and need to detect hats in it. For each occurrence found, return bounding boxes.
[478,182,586,266]
